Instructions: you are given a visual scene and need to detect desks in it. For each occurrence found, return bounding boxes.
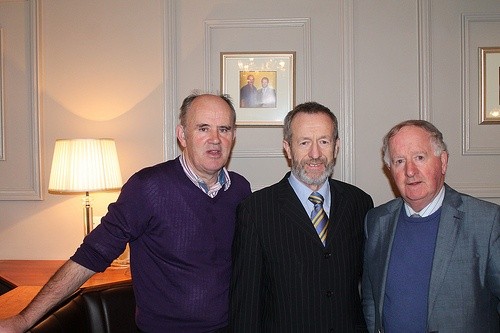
[0,260,132,320]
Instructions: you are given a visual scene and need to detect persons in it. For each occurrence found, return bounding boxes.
[361,119,500,332]
[228,101,374,332]
[240,74,276,107]
[0,90,252,333]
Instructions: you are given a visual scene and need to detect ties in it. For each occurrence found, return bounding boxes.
[308,191,330,246]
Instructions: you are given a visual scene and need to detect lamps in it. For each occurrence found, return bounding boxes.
[46,139,123,237]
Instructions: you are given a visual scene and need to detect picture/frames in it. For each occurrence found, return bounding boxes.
[477,47,500,125]
[219,51,297,128]
[0,26,8,160]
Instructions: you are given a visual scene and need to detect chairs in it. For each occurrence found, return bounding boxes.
[82,285,137,333]
[24,294,89,333]
